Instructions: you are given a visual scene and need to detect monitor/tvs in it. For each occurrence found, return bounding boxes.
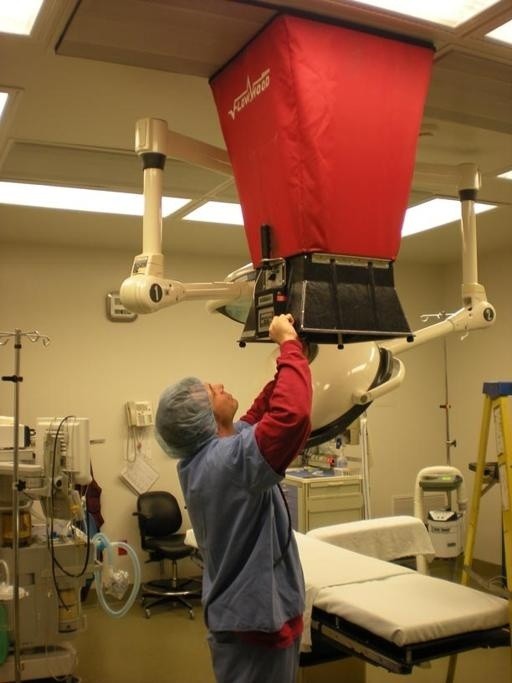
[34,416,92,487]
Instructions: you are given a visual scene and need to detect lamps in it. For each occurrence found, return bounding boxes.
[217,261,258,324]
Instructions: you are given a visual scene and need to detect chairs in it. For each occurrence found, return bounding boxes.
[137,491,202,620]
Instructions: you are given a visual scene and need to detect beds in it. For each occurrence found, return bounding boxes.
[185,528,509,675]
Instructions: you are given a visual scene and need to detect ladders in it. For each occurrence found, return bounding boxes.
[462,382,512,599]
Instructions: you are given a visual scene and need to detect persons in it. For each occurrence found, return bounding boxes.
[148,314,311,682]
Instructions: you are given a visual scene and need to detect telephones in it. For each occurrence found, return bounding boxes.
[125,401,153,428]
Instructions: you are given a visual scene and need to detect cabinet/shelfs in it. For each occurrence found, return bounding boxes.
[277,467,364,534]
